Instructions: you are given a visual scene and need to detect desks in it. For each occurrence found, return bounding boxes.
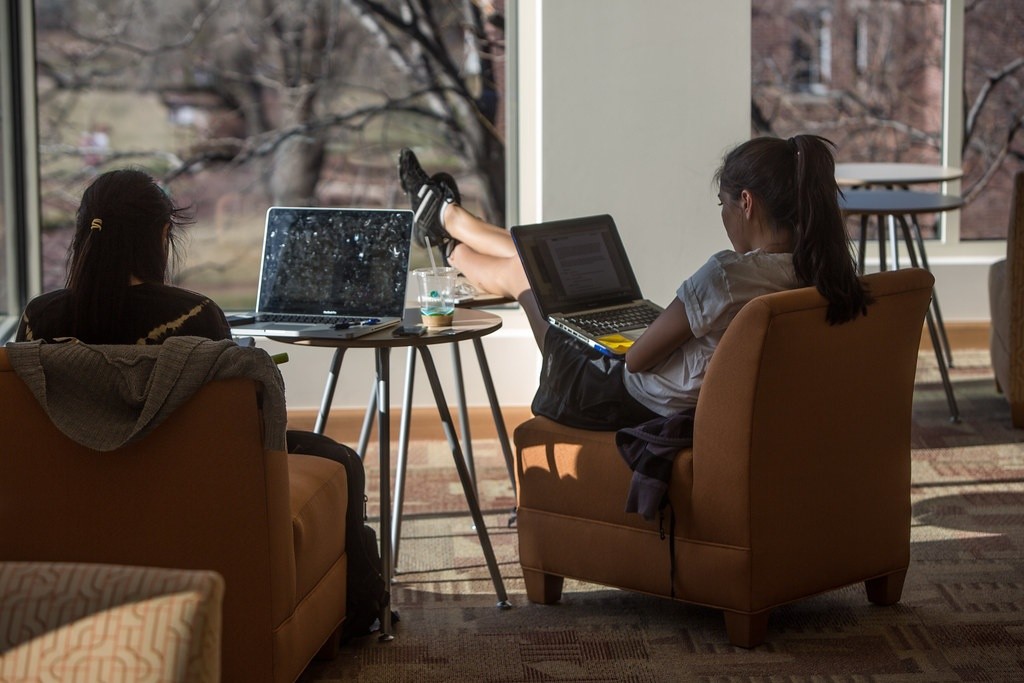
[357,293,518,531]
[265,304,512,642]
[834,161,963,368]
[835,188,966,424]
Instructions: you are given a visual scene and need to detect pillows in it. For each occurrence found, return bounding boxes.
[1,591,202,683]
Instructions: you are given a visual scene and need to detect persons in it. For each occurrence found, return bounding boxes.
[8,168,234,344]
[398,135,878,431]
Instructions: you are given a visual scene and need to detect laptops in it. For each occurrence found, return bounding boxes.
[228,207,415,338]
[510,214,668,360]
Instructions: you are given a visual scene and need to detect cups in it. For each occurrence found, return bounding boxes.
[412,267,460,328]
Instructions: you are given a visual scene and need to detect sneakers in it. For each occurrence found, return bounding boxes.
[430,171,463,278]
[399,146,456,248]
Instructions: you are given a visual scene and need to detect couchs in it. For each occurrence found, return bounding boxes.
[0,562,223,683]
[989,169,1024,431]
[515,268,936,649]
[0,342,348,683]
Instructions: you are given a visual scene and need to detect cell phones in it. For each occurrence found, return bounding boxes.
[225,315,255,327]
[391,325,428,338]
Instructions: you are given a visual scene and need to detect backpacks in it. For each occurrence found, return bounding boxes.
[286,429,386,641]
